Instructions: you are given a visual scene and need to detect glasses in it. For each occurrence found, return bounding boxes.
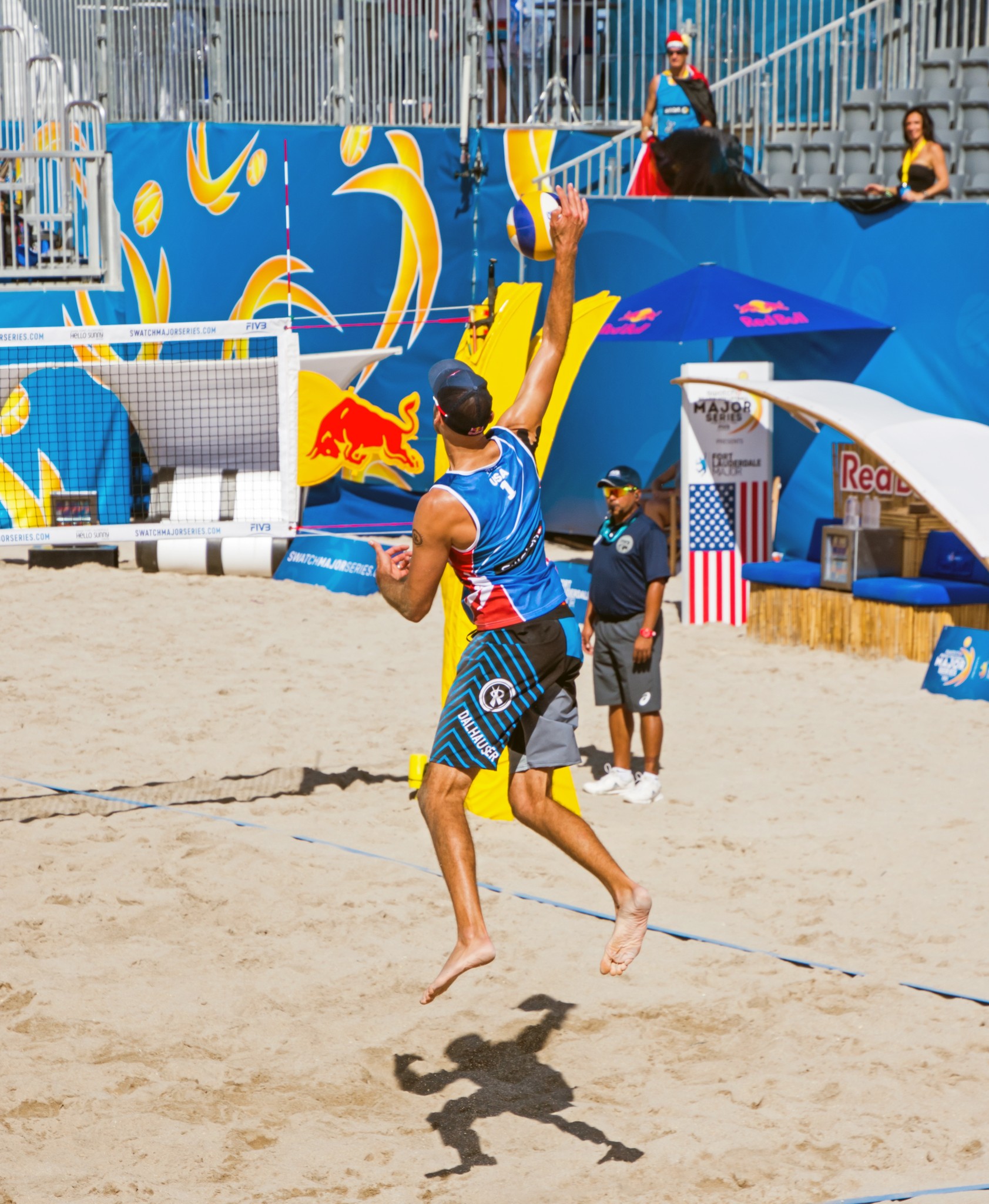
[602,487,638,497]
[667,49,684,55]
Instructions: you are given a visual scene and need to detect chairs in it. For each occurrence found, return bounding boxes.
[764,44,989,205]
[636,476,782,582]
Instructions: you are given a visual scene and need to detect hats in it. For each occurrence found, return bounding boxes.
[667,32,684,48]
[598,465,641,488]
[428,359,493,436]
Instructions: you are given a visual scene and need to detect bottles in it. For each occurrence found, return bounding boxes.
[843,495,880,529]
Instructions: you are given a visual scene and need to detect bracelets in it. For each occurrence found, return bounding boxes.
[641,127,650,131]
[921,190,928,199]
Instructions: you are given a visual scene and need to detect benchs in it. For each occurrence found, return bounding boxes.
[742,518,989,664]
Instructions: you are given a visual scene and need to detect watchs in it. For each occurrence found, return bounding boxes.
[639,628,657,638]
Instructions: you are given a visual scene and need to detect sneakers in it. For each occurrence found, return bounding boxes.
[622,771,662,803]
[583,763,635,795]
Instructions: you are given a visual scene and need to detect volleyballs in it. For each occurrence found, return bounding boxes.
[506,191,566,262]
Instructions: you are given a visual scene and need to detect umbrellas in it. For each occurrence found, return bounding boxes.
[593,263,895,361]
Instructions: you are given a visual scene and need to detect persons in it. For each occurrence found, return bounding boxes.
[864,105,951,202]
[366,182,653,1007]
[580,465,669,803]
[639,31,717,141]
